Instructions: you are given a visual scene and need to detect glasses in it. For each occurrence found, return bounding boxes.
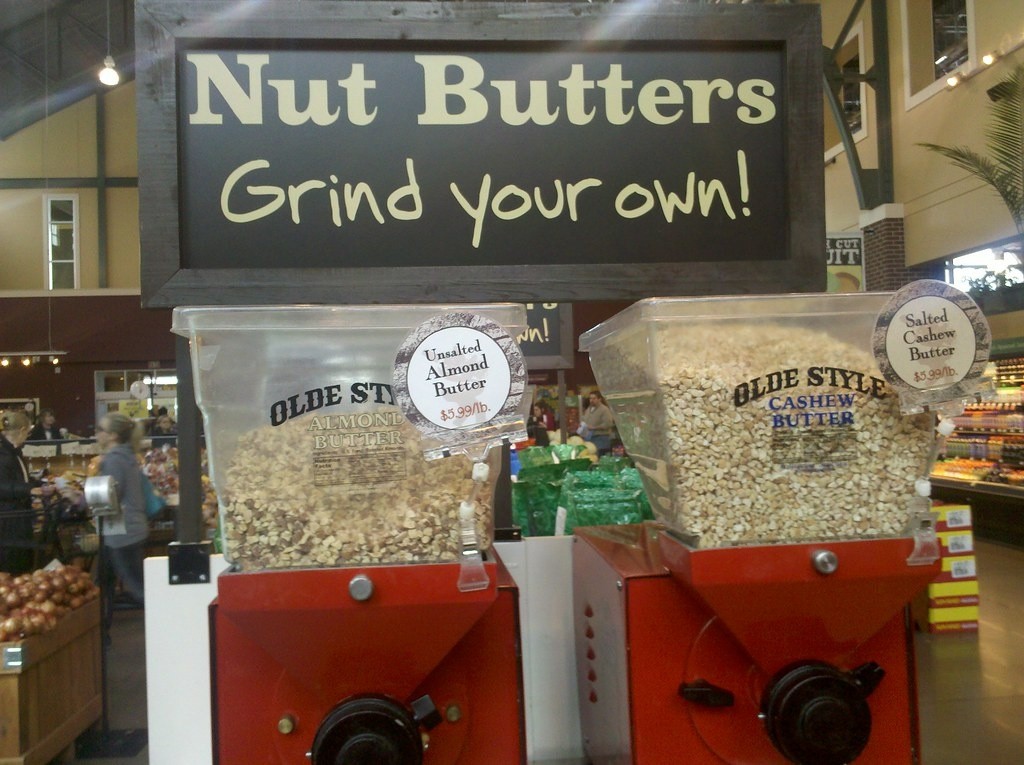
[94,425,112,433]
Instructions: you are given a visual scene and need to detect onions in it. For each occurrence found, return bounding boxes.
[0,566,98,643]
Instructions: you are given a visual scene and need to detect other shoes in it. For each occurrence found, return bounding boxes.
[105,635,112,649]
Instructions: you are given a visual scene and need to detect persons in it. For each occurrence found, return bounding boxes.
[583,391,613,456]
[31,407,64,440]
[150,415,177,448]
[0,411,55,577]
[94,411,150,651]
[534,400,555,447]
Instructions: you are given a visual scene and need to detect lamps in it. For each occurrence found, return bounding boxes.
[49,355,59,364]
[21,355,30,366]
[97,0,121,86]
[983,50,1000,64]
[1,356,9,366]
[947,71,964,87]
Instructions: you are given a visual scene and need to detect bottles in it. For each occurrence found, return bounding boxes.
[933,358,1024,486]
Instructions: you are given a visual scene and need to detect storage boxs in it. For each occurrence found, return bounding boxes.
[927,503,980,634]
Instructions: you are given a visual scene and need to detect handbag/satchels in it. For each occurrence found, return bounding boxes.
[139,470,165,517]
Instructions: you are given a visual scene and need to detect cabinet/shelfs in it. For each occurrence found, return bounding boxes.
[927,351,1024,548]
